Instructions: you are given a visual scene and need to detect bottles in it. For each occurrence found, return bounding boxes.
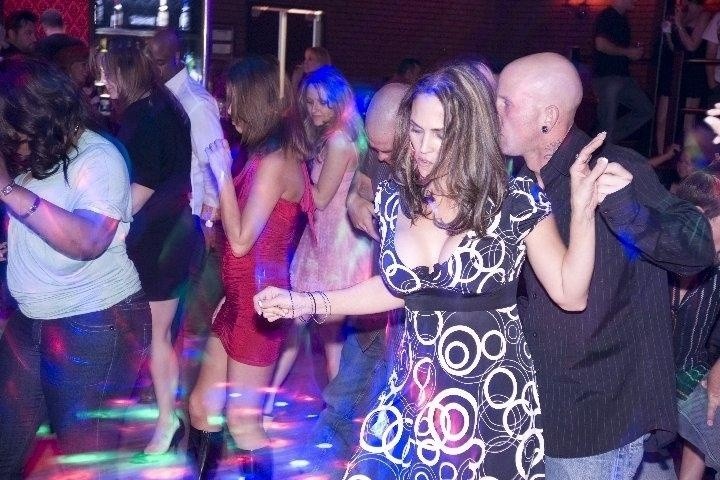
[177,1,192,31]
[109,1,124,29]
[93,1,105,28]
[154,0,170,29]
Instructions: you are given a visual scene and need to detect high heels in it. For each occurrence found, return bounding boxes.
[136,416,185,458]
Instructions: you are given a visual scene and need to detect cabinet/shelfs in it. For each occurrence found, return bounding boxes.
[91,0,202,51]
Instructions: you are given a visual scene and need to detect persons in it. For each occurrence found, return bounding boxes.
[1,1,719,480]
